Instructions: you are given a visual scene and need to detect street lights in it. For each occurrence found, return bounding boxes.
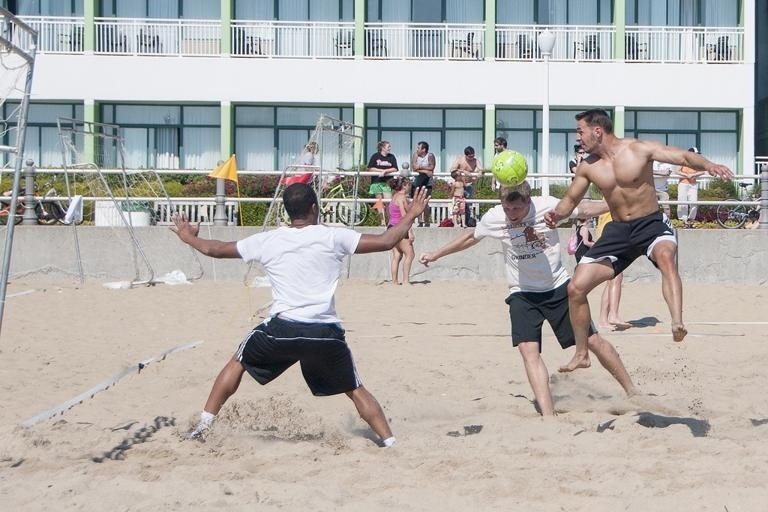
[535,26,555,197]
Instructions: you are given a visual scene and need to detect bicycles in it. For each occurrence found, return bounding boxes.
[1,173,73,226]
[280,167,369,228]
[715,182,767,228]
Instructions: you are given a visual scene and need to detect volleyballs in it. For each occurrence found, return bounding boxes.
[490,151,527,186]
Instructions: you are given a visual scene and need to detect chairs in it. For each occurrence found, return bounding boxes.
[57,24,737,60]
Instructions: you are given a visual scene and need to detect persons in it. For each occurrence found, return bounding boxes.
[449,147,481,227]
[677,145,704,230]
[569,216,595,268]
[384,175,419,289]
[446,172,467,228]
[167,182,431,447]
[491,137,509,191]
[367,141,397,229]
[300,142,319,181]
[420,181,644,422]
[590,194,633,330]
[544,108,733,373]
[571,144,591,187]
[411,141,435,203]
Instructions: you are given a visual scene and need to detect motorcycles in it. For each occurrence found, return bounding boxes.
[0,182,62,225]
